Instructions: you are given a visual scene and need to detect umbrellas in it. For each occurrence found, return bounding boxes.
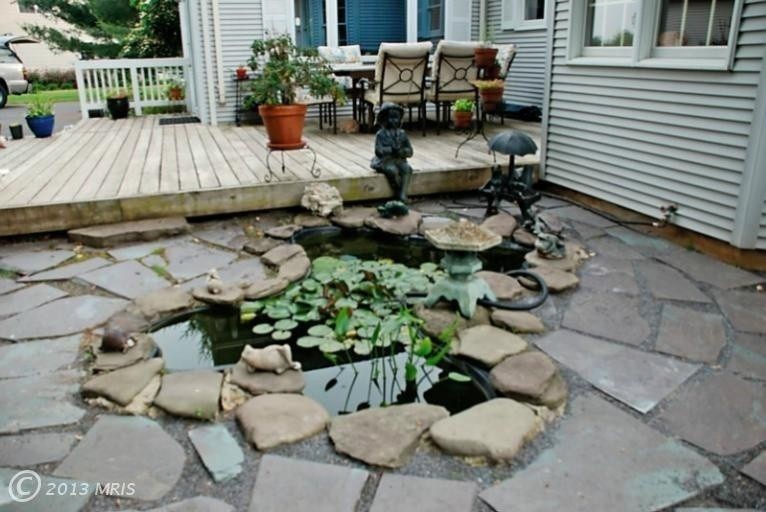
[486,129,538,179]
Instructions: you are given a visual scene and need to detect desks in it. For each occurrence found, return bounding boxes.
[310,60,432,129]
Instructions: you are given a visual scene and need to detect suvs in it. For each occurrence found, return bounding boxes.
[1,34,42,108]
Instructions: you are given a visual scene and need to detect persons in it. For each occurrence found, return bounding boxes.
[478,163,535,195]
[370,100,414,204]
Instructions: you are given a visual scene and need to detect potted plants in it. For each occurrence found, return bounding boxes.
[8,90,56,139]
[243,28,350,149]
[104,85,132,120]
[163,80,183,102]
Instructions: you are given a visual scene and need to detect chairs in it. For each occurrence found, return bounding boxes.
[267,38,518,139]
